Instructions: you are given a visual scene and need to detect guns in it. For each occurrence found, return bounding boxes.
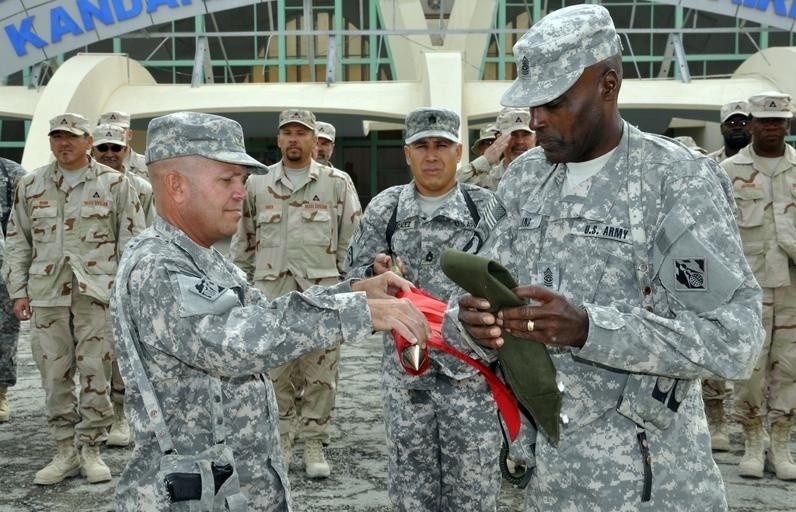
[164,464,233,502]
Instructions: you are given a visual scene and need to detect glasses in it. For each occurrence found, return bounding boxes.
[483,140,493,145]
[97,145,125,152]
[723,119,751,128]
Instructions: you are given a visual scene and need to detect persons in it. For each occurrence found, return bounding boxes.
[672,95,796,480]
[340,108,505,512]
[438,3,768,512]
[227,109,362,478]
[455,110,538,193]
[109,112,434,511]
[0,112,155,485]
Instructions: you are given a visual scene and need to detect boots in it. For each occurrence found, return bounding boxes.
[739,417,766,479]
[281,439,294,474]
[704,400,733,450]
[79,443,113,485]
[765,417,796,480]
[303,437,330,478]
[106,401,131,446]
[0,382,10,423]
[33,438,81,485]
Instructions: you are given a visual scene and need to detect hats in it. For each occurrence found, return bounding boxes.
[720,100,750,127]
[496,107,536,136]
[99,111,131,128]
[749,91,794,118]
[278,109,317,135]
[92,124,127,146]
[48,113,92,138]
[500,4,624,107]
[315,121,336,143]
[144,111,271,175]
[471,124,499,157]
[403,107,460,144]
